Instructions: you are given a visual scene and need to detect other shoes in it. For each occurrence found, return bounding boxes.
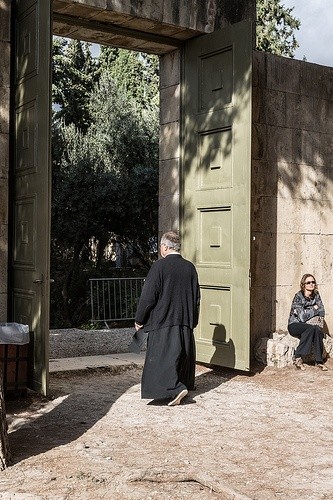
[295,359,305,370]
[316,363,328,371]
[168,389,188,406]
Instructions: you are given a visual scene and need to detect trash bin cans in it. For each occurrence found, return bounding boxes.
[0,319,33,414]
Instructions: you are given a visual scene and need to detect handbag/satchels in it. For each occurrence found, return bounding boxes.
[306,316,325,328]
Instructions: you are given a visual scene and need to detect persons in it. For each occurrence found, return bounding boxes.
[135,231,201,406]
[287,274,329,371]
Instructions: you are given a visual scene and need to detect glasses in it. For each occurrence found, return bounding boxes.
[158,243,164,248]
[303,281,315,284]
